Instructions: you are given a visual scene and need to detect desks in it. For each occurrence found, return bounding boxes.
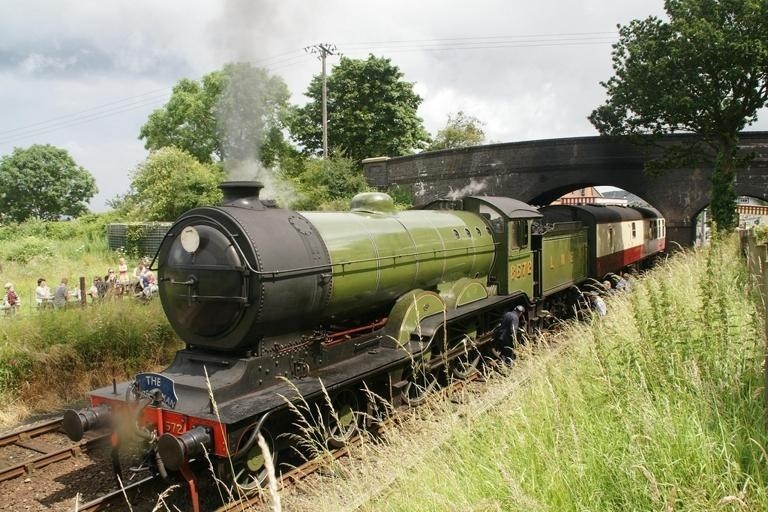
[117,281,136,294]
[39,297,54,304]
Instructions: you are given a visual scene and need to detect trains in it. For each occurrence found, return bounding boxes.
[61,179,666,497]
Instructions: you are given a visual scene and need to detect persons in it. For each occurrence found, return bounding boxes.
[616,273,629,287]
[54,277,71,307]
[35,278,51,305]
[583,289,606,317]
[493,304,526,364]
[602,280,615,297]
[69,257,158,304]
[3,281,21,308]
